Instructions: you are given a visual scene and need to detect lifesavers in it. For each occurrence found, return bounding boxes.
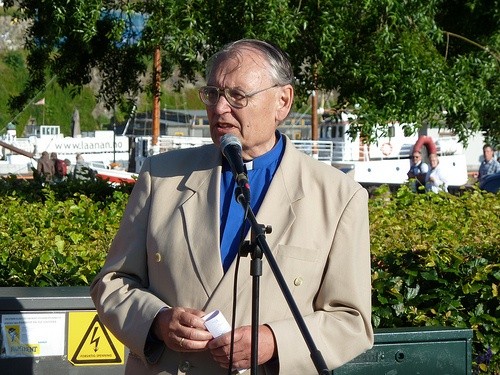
[381,143,393,157]
[412,135,440,156]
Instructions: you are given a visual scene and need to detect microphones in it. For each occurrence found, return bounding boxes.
[220,134,251,204]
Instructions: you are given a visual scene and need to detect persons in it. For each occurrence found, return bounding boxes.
[89,39,374,375]
[407,151,429,194]
[477,144,500,195]
[425,154,449,194]
[110,160,120,169]
[36,151,95,184]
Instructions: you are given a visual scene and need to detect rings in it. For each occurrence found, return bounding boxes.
[179,338,184,348]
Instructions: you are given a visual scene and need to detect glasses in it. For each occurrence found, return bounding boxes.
[198,85,279,108]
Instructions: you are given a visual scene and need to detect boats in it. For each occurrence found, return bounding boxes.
[0,44,500,193]
